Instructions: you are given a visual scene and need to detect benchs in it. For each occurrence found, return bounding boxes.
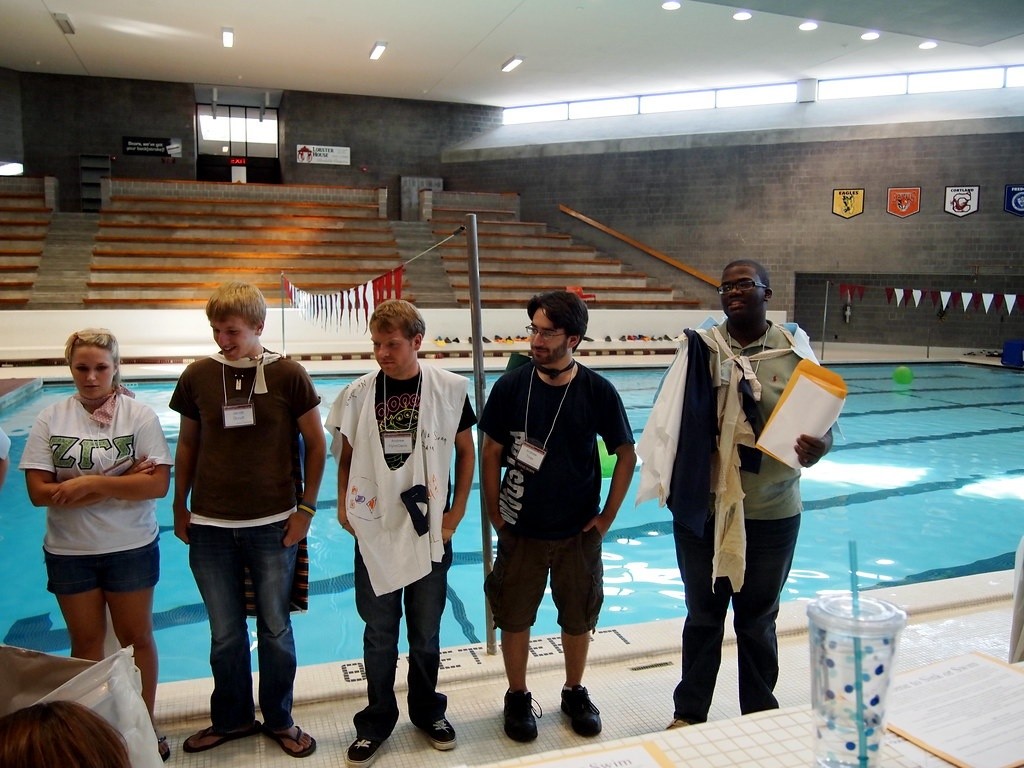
[0,192,53,307]
[82,194,415,310]
[427,207,699,309]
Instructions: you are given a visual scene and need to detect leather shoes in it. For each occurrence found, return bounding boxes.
[504,688,542,742]
[561,685,602,737]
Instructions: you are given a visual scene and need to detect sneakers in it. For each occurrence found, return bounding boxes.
[344,737,386,768]
[420,716,457,751]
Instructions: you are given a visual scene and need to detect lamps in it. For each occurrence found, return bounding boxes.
[501,55,523,72]
[221,27,233,48]
[370,41,388,60]
[54,13,76,35]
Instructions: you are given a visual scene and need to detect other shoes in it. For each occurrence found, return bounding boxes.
[666,718,690,730]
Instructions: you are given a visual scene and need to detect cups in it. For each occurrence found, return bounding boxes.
[806,594,908,768]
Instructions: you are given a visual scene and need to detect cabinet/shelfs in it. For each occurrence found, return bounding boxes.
[78,153,111,213]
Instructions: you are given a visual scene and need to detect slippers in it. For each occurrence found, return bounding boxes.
[183,720,261,753]
[261,722,317,758]
[157,735,171,762]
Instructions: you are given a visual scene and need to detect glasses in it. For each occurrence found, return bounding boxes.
[525,325,569,340]
[716,280,768,295]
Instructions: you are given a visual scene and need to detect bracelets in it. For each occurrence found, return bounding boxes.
[298,503,316,516]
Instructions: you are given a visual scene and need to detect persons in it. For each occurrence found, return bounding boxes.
[666,259,834,731]
[18,327,174,762]
[0,701,131,768]
[168,281,327,758]
[477,290,637,742]
[325,298,478,768]
